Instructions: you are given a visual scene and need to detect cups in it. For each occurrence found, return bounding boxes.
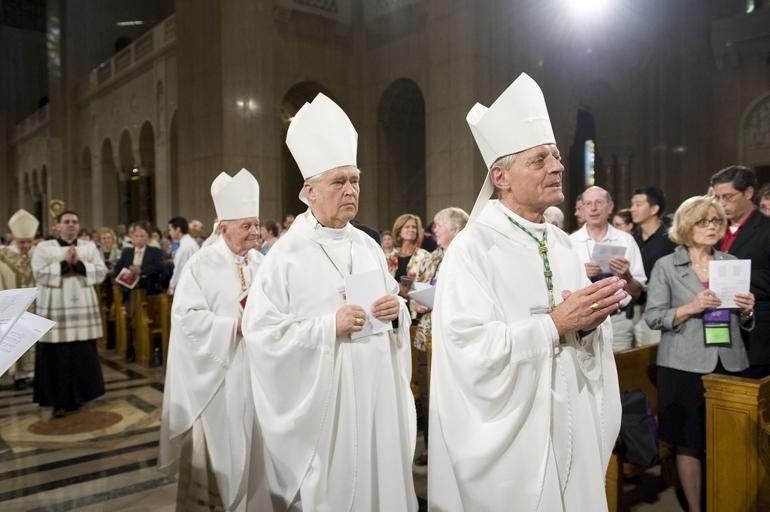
[400,275,412,293]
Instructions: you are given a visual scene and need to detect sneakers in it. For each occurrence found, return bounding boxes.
[52,405,82,417]
[14,377,33,390]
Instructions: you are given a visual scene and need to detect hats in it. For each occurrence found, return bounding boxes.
[210,167,260,221]
[466,72,558,168]
[285,92,359,180]
[9,209,40,238]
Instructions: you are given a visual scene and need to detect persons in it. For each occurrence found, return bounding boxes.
[542,161,770,511]
[257,204,473,466]
[424,70,629,511]
[159,167,277,511]
[1,208,218,418]
[242,91,422,511]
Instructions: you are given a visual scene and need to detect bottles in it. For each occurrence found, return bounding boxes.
[155,347,161,365]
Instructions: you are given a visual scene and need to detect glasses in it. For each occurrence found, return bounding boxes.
[696,218,722,227]
[715,193,735,202]
